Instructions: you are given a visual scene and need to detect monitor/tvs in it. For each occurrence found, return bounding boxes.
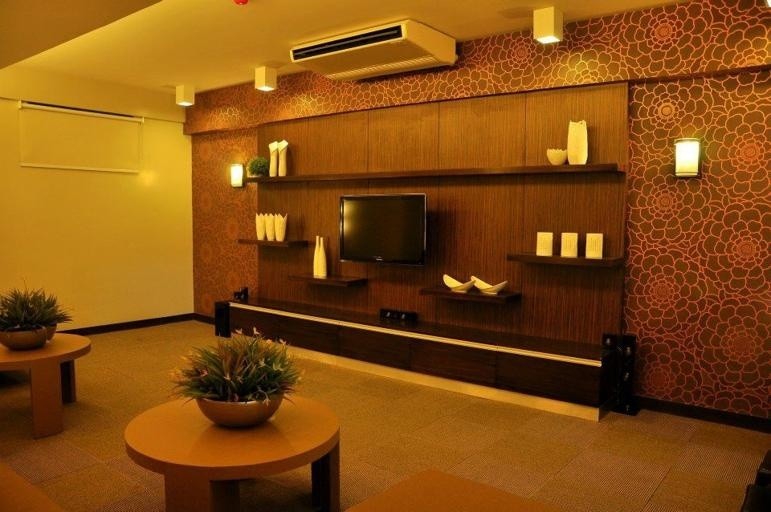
[339,193,427,266]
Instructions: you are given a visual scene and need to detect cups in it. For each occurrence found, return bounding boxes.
[568,120,590,166]
[254,211,290,242]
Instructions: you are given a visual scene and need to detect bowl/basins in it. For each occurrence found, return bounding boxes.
[547,148,569,166]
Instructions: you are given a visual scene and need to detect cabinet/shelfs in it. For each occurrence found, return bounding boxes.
[228,295,606,421]
[239,233,368,290]
[417,239,625,307]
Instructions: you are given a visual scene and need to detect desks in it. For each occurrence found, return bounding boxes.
[124,391,343,511]
[0,333,92,436]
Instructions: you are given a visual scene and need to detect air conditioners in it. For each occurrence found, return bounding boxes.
[289,17,459,82]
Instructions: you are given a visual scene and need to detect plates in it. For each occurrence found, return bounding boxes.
[443,273,477,293]
[470,275,509,296]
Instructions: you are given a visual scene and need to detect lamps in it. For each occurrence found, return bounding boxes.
[532,5,563,46]
[172,82,196,106]
[230,160,245,189]
[252,65,279,91]
[672,135,704,179]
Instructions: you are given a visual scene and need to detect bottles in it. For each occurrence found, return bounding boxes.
[268,139,290,177]
[314,236,328,280]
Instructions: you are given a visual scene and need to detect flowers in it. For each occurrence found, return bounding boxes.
[172,325,310,406]
[0,278,73,329]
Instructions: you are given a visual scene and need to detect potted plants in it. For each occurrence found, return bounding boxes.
[246,155,269,179]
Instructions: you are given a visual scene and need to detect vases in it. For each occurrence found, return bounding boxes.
[193,395,283,428]
[312,234,320,278]
[267,139,279,178]
[0,324,55,349]
[318,237,328,277]
[277,141,290,178]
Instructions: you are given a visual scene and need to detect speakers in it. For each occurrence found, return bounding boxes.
[215,301,231,338]
[601,332,640,417]
